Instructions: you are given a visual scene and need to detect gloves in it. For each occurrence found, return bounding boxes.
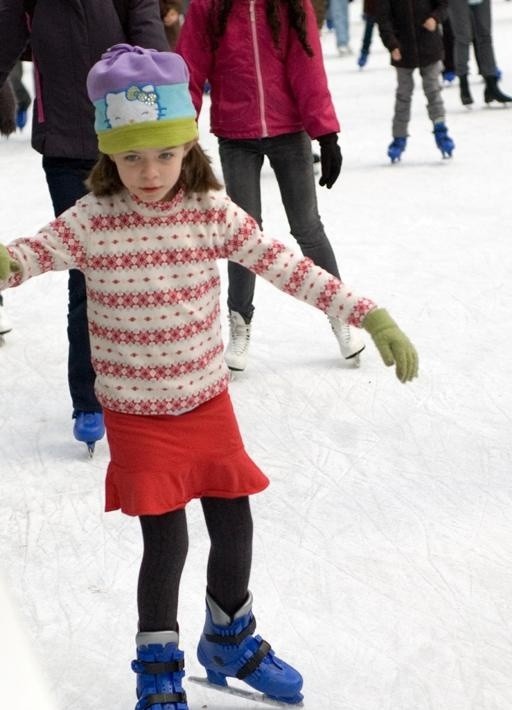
[0,243,22,280]
[361,308,418,383]
[318,132,343,191]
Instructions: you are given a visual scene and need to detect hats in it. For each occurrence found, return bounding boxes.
[86,43,199,155]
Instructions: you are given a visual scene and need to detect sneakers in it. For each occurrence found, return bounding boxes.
[358,50,368,67]
[442,69,456,82]
[71,410,105,444]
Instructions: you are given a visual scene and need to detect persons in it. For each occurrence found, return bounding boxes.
[168,0,367,370]
[0,43,420,709]
[307,0,512,166]
[3,61,30,137]
[2,0,174,442]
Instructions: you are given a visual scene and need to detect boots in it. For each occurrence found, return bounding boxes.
[198,594,304,698]
[483,75,512,103]
[387,137,406,158]
[433,123,454,152]
[326,313,365,359]
[131,626,186,710]
[459,74,473,104]
[225,310,252,371]
